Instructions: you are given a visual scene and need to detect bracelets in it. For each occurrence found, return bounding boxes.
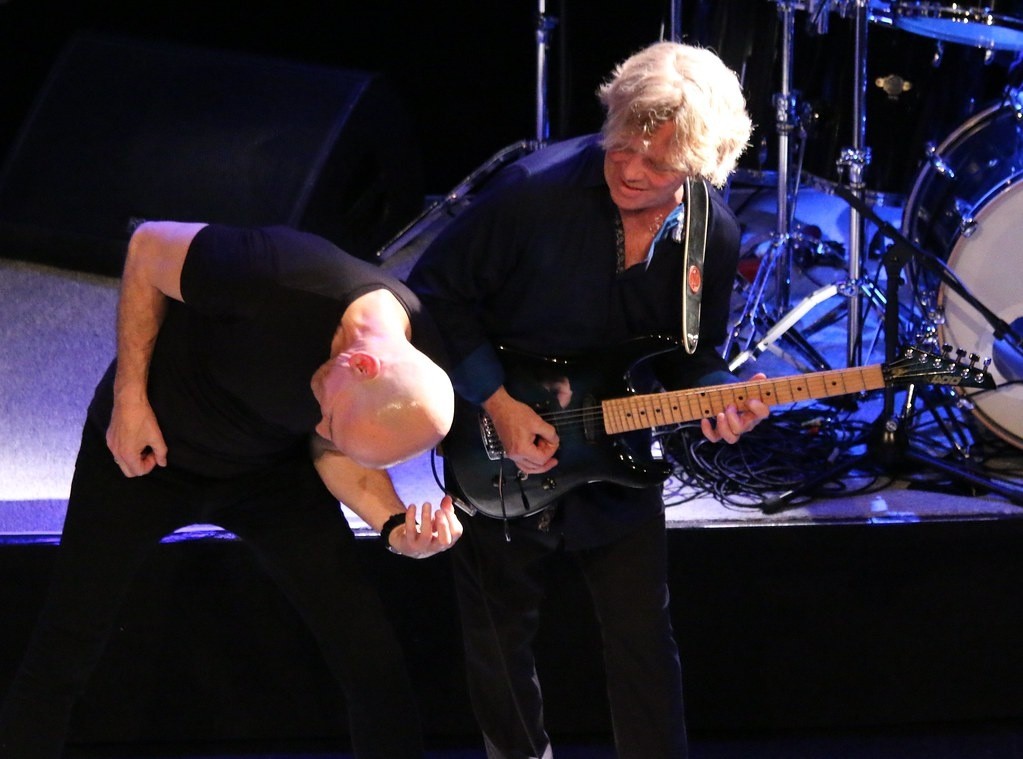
[381,512,419,555]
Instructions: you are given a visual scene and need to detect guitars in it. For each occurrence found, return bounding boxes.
[441,332,996,519]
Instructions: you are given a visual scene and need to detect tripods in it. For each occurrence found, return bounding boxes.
[718,0,1023,517]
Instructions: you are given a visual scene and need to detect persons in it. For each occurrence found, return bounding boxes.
[54,222,465,759]
[406,42,772,759]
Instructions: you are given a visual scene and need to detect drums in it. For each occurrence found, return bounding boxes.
[899,90,1023,455]
[728,1,1023,198]
